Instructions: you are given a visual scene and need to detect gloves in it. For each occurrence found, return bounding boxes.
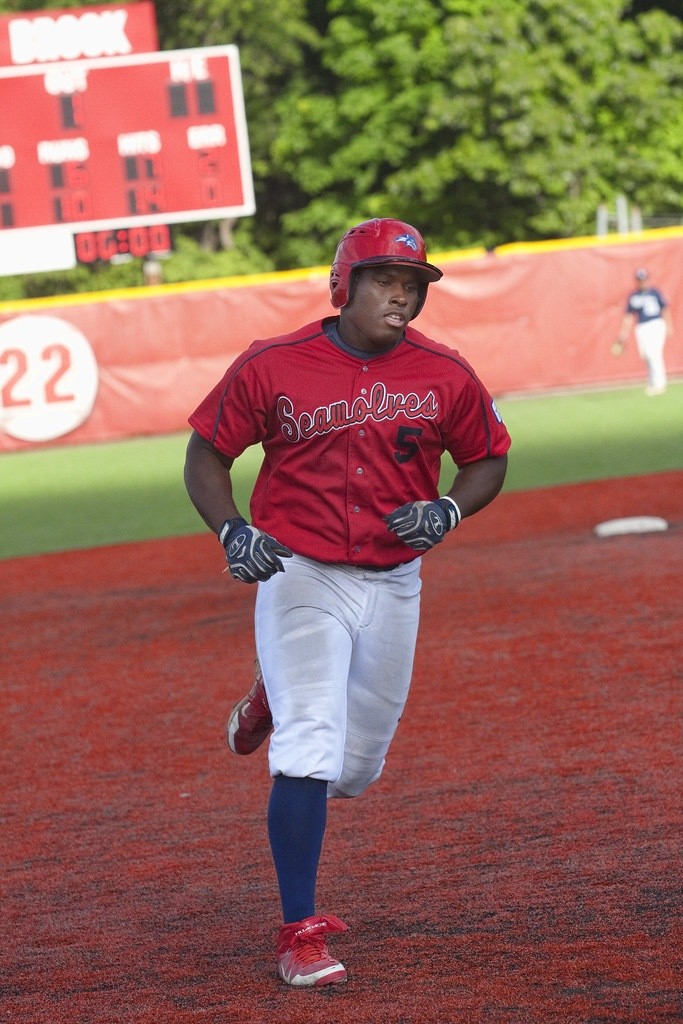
[217,517,294,586]
[381,495,463,552]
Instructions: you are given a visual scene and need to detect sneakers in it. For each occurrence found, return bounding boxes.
[275,912,350,986]
[227,653,275,755]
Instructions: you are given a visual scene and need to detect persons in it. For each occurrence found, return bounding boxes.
[185,226,511,986]
[613,268,673,394]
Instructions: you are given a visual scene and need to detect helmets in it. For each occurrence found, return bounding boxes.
[329,218,444,322]
